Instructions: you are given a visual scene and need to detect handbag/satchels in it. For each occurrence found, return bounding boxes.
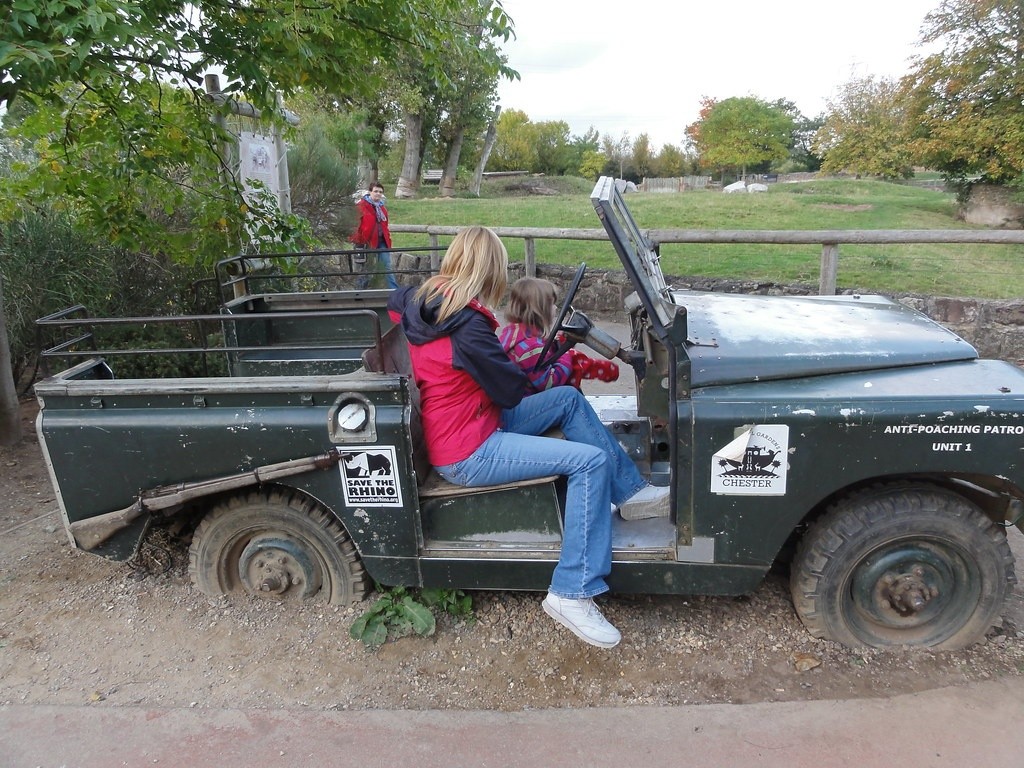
[353,244,367,264]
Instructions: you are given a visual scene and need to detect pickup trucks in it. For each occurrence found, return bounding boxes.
[33,176,1023,651]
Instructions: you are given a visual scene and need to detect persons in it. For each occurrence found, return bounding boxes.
[388,225,671,649]
[349,182,400,289]
[498,278,619,393]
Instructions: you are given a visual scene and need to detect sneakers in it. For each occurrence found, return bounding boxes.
[542,592,621,648]
[620,484,670,521]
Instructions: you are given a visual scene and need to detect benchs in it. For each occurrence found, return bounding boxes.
[423,170,443,184]
[361,324,565,538]
